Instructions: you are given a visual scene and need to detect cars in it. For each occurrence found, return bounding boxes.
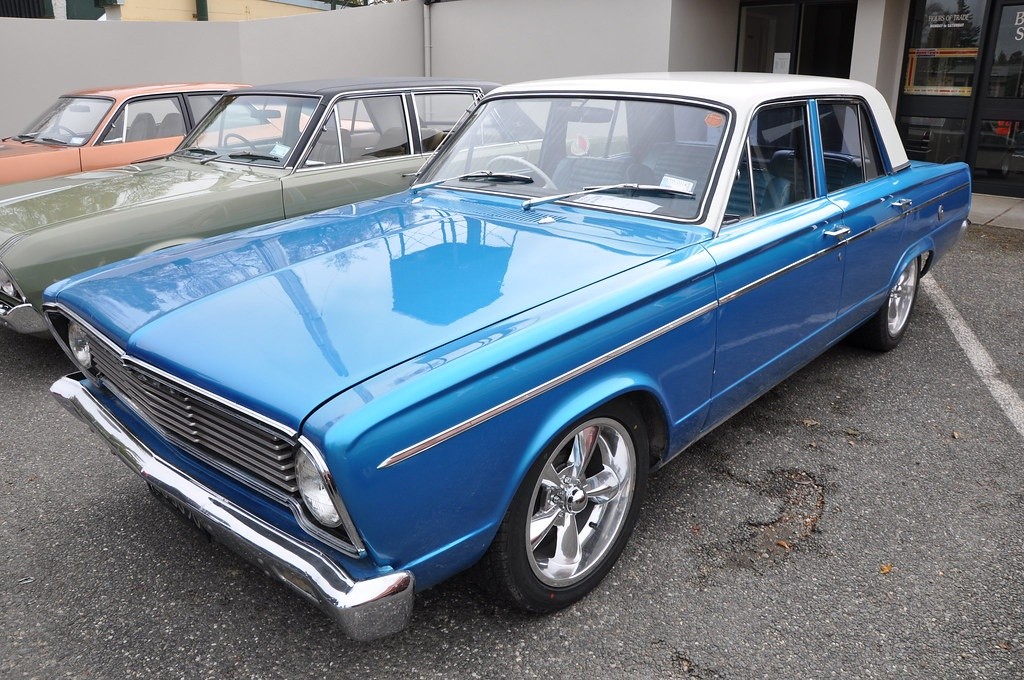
[0,71,974,643]
[905,117,1018,178]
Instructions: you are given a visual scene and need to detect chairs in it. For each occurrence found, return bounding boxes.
[375,128,443,151]
[308,128,351,166]
[551,140,865,222]
[125,113,186,142]
